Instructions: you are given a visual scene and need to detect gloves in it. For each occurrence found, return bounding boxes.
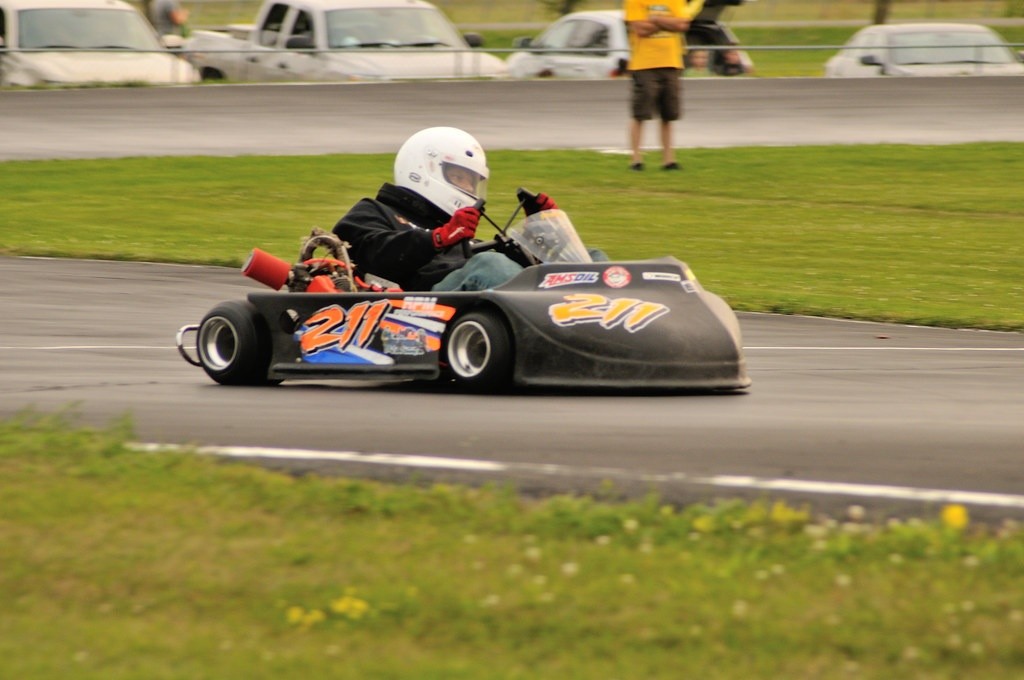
[432,207,480,251]
[524,193,558,217]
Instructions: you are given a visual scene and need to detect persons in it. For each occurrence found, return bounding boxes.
[145,1,190,36]
[622,0,691,171]
[331,126,610,292]
[682,50,717,76]
[713,43,750,77]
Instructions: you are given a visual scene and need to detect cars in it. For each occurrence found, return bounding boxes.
[822,18,1024,78]
[0,0,202,86]
[504,0,754,79]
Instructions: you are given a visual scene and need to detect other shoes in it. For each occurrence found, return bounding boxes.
[659,162,678,171]
[628,163,644,171]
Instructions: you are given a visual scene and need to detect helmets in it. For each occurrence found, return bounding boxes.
[393,126,488,217]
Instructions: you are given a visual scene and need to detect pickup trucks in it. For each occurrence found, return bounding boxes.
[186,0,515,82]
